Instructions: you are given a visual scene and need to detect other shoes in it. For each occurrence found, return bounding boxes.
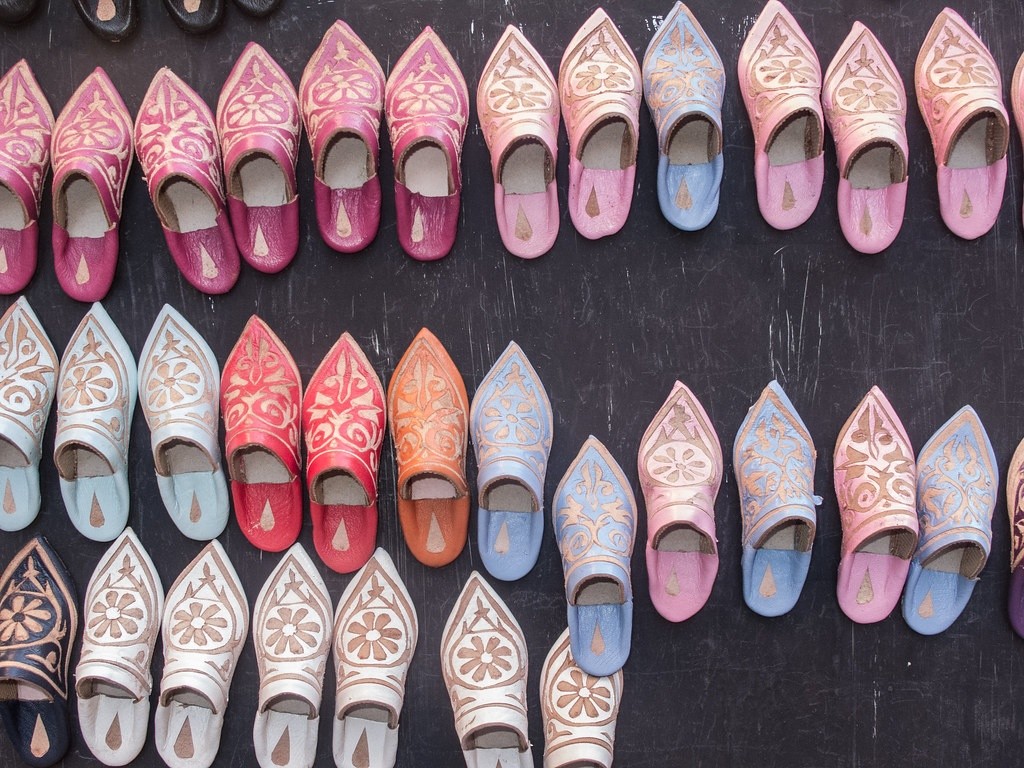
[559,6,643,240]
[384,24,470,261]
[0,58,55,296]
[169,0,228,39]
[132,66,241,294]
[237,0,282,19]
[0,0,46,31]
[0,290,1023,768]
[641,0,726,232]
[737,1,825,231]
[823,20,909,255]
[76,0,140,45]
[298,18,387,254]
[215,42,302,275]
[1010,53,1023,150]
[50,66,135,303]
[914,6,1010,241]
[476,23,561,261]
